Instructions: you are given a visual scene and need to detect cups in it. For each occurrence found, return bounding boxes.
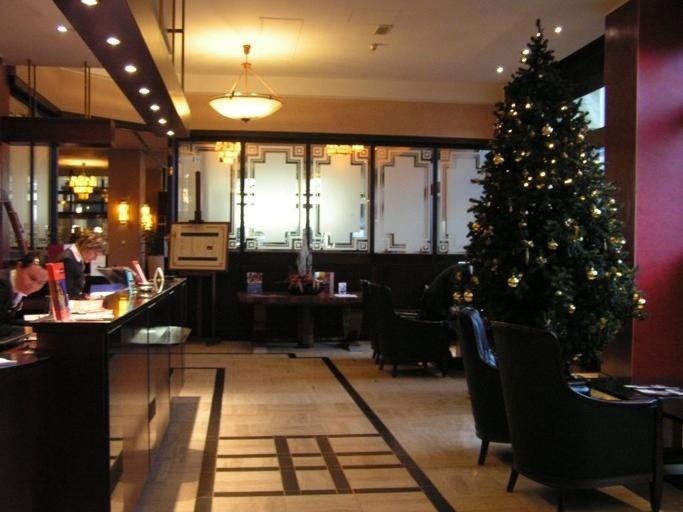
[339,282,347,295]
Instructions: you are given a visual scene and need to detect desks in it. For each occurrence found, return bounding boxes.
[0,333,45,511]
[580,377,683,492]
[235,291,364,348]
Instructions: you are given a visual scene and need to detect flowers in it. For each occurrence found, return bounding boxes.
[285,273,320,292]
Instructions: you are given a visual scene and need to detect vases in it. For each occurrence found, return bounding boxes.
[288,283,326,295]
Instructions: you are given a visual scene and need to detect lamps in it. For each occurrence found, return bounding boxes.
[144,216,153,231]
[209,44,284,124]
[215,141,241,163]
[326,144,353,171]
[68,164,98,201]
[118,200,130,225]
[141,202,150,225]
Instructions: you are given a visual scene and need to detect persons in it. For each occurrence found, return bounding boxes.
[0,249,49,339]
[43,232,108,300]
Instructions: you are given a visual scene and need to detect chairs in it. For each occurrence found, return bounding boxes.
[460,307,586,466]
[490,320,664,512]
